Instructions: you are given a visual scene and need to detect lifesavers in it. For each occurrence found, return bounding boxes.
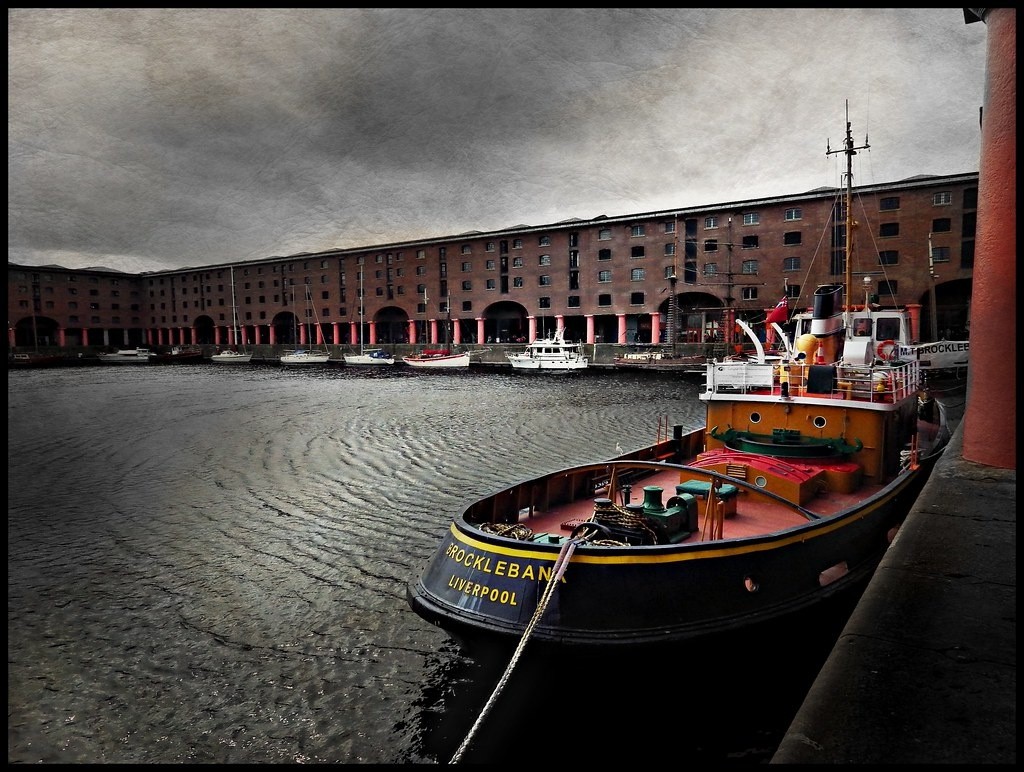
[878,340,898,360]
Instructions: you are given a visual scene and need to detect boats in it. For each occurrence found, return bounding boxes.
[98,346,151,364]
[402,96,957,670]
[148,345,205,365]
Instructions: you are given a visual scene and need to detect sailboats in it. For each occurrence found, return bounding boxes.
[280,285,333,365]
[345,266,396,366]
[209,266,253,363]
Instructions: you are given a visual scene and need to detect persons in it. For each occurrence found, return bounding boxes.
[704,334,714,360]
[486,334,527,343]
[635,333,642,343]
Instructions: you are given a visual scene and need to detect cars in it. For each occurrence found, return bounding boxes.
[9,350,54,361]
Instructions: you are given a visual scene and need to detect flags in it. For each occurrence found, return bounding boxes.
[766,296,788,323]
